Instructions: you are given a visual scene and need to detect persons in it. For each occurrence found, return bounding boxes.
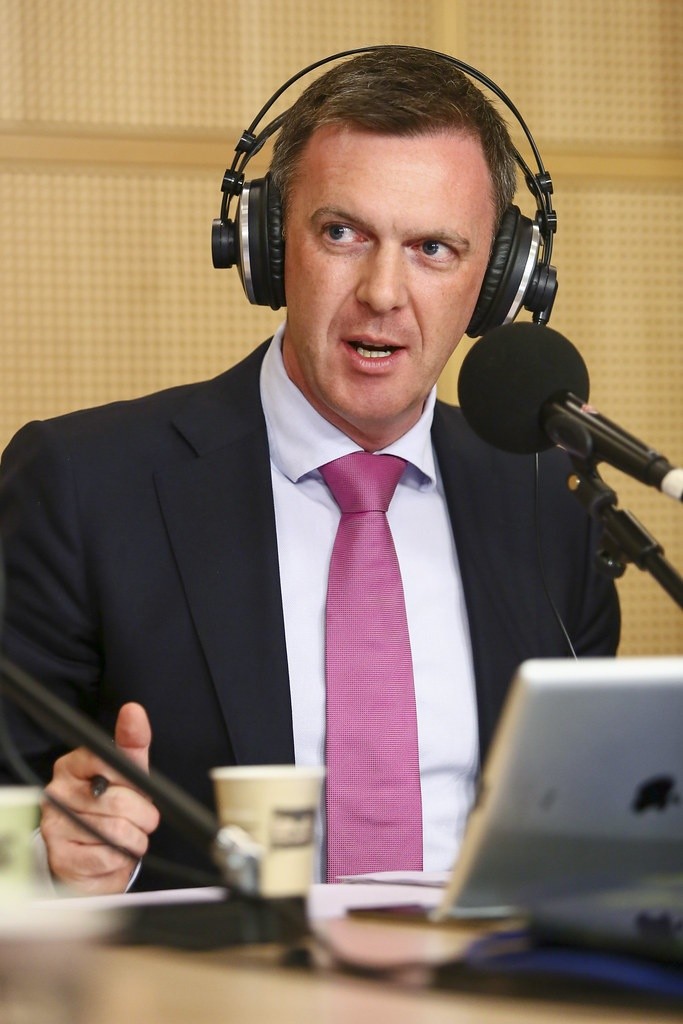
[0,45,621,956]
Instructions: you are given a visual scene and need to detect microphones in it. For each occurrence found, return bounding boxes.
[459,321,683,506]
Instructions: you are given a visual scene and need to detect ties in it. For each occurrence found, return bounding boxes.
[318,456,425,885]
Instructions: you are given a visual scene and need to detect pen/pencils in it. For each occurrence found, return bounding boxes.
[85,776,112,801]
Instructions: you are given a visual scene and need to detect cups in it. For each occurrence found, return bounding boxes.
[209,764,331,908]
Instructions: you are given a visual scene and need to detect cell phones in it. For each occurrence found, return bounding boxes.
[348,904,530,934]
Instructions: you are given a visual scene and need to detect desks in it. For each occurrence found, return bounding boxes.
[0,877,682,1024]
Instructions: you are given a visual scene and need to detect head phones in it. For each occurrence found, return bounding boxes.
[210,46,556,339]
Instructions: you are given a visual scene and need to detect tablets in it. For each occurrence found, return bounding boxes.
[441,656,683,912]
